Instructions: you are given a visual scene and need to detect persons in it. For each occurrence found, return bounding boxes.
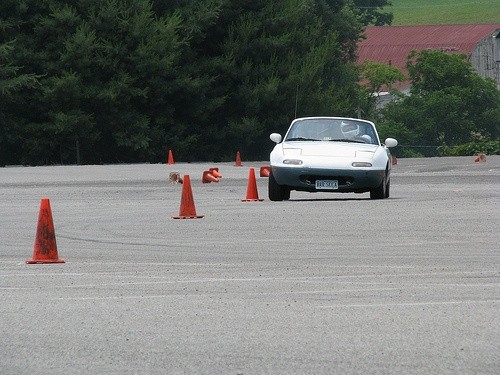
[340,121,359,138]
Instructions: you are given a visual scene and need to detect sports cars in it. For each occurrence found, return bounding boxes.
[267,116,398,202]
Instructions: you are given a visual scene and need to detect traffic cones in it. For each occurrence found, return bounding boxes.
[259,166,270,177]
[169,172,183,184]
[25,197,66,264]
[172,174,205,220]
[167,149,175,165]
[241,168,264,202]
[474,154,486,163]
[233,151,244,166]
[208,167,222,178]
[391,156,397,165]
[202,170,219,183]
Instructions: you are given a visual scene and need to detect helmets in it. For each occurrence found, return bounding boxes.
[340,121,359,138]
